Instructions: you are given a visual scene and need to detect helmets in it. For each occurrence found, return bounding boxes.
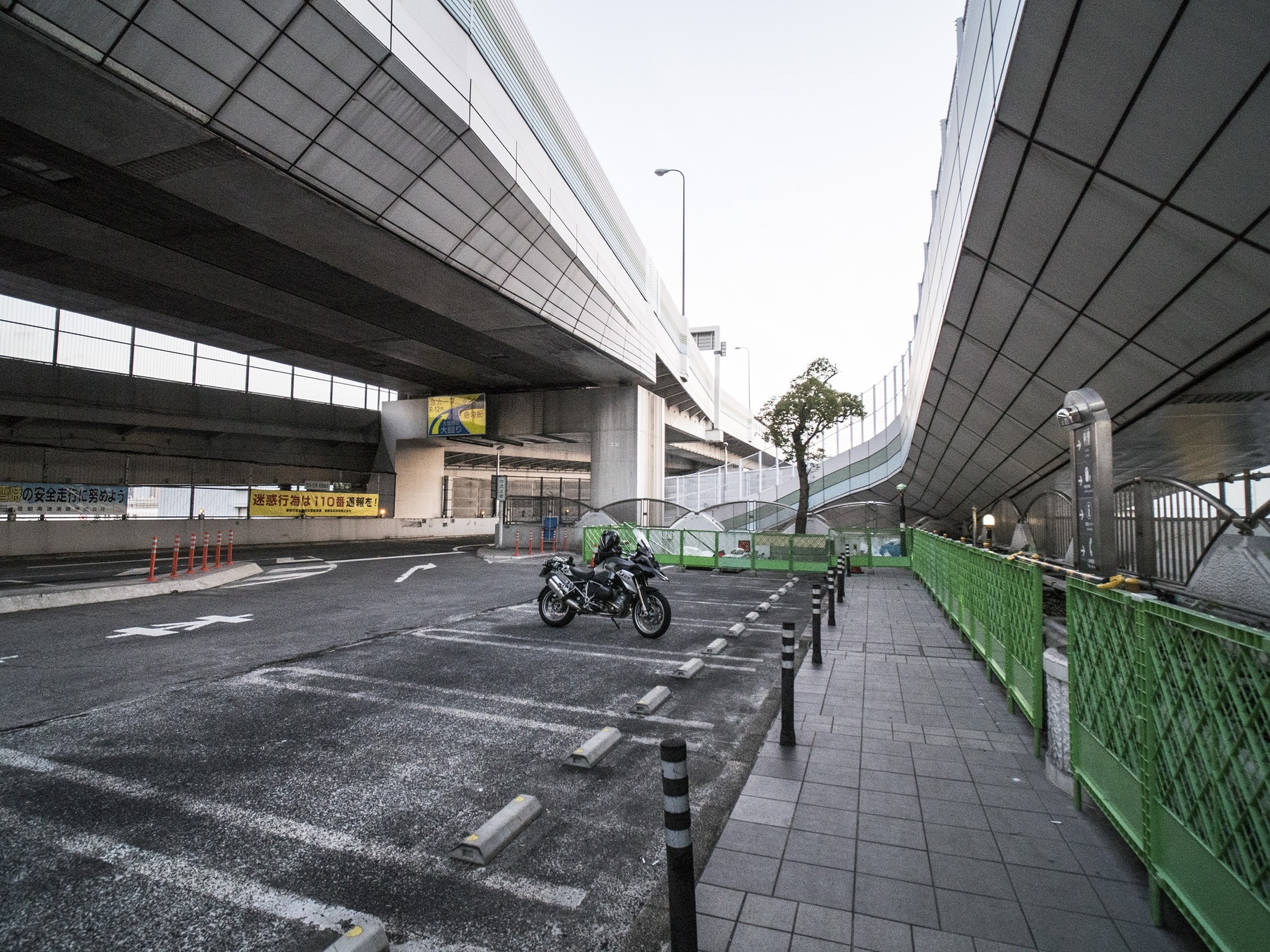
[600,529,620,551]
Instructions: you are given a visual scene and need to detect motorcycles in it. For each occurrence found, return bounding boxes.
[537,521,671,639]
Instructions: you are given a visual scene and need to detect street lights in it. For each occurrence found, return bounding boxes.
[982,513,996,550]
[654,168,685,316]
[735,346,750,408]
[896,483,908,557]
